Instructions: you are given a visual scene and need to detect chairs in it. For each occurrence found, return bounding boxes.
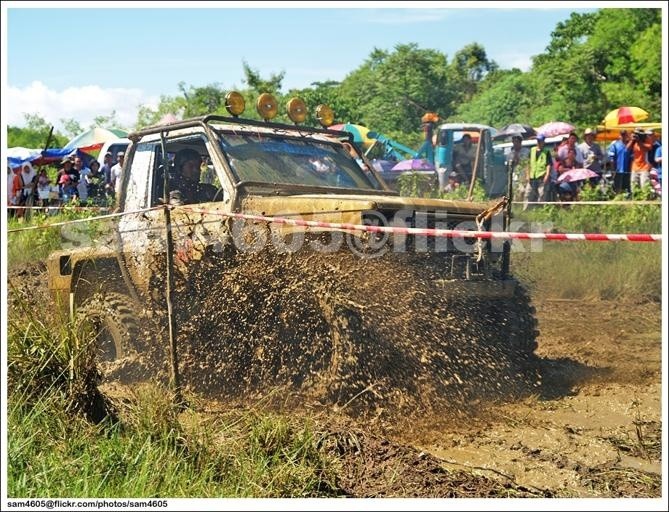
[154,164,178,205]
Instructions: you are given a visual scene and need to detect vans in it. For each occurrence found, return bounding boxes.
[490,134,572,158]
[96,137,133,171]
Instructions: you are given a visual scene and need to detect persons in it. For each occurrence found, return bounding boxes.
[159,148,223,204]
[505,127,662,211]
[7,151,125,220]
[453,133,477,184]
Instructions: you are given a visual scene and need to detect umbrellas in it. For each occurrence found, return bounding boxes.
[603,106,649,126]
[537,120,575,148]
[494,123,536,141]
[327,121,378,146]
[62,125,129,151]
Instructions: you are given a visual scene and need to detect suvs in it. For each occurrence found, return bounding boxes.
[49,91,538,411]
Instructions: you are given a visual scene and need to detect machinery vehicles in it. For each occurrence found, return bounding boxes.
[367,112,508,197]
